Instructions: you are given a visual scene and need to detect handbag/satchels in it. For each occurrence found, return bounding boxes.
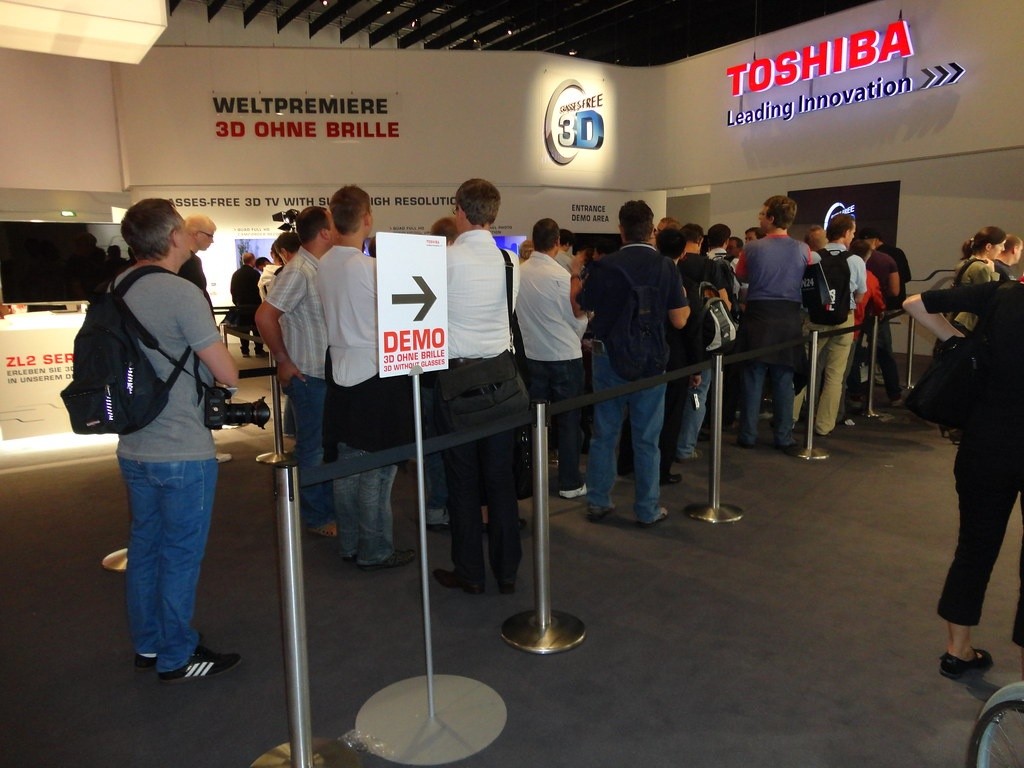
[904,281,1013,430]
[437,348,528,433]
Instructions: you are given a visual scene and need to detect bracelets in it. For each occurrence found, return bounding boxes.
[571,274,580,281]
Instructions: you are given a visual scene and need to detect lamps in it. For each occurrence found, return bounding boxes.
[411,17,422,29]
[568,40,578,56]
[270,207,299,233]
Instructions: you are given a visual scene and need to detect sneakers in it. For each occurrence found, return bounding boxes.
[159,647,243,685]
[135,652,157,671]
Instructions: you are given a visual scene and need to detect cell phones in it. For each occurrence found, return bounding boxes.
[590,339,604,354]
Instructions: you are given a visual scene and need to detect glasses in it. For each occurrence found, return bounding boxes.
[453,206,457,214]
[199,230,213,239]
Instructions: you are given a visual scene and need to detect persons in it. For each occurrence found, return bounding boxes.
[952,225,1024,330]
[571,199,690,527]
[230,232,301,437]
[427,218,526,532]
[514,194,912,499]
[2,232,136,303]
[255,205,336,536]
[315,185,417,571]
[432,179,521,596]
[107,198,240,682]
[178,214,231,464]
[902,277,1024,680]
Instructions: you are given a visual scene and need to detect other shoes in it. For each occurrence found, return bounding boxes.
[559,481,587,498]
[340,552,358,562]
[588,501,617,520]
[256,350,270,358]
[941,649,993,679]
[850,393,861,410]
[660,473,681,485]
[482,517,528,533]
[890,396,902,407]
[642,508,668,525]
[307,520,338,537]
[499,583,513,593]
[433,568,482,595]
[360,549,417,571]
[675,449,704,461]
[425,506,451,525]
[242,353,249,357]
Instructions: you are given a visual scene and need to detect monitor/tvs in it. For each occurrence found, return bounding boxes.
[1,221,137,305]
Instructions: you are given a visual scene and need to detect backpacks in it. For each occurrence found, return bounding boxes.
[801,248,856,326]
[63,266,191,436]
[602,253,670,382]
[697,281,736,354]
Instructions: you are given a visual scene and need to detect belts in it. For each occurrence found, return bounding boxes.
[444,357,483,368]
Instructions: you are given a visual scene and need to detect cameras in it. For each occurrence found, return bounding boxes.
[205,387,270,430]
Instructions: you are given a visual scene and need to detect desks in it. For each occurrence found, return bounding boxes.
[0,313,87,440]
